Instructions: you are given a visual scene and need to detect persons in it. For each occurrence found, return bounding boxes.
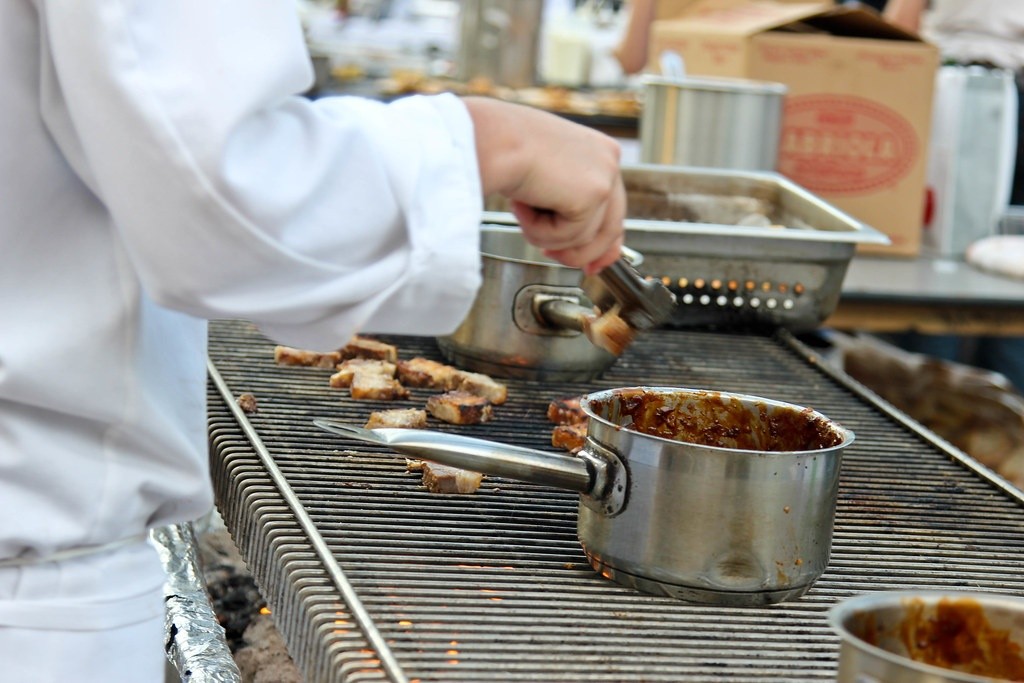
[1,1,627,683]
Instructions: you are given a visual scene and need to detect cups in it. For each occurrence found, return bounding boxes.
[923,65,1017,259]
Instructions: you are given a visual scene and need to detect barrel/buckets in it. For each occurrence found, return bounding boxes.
[637,74,788,173]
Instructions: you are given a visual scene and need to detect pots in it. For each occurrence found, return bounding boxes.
[829,591,1024,683]
[436,224,643,383]
[312,385,857,605]
